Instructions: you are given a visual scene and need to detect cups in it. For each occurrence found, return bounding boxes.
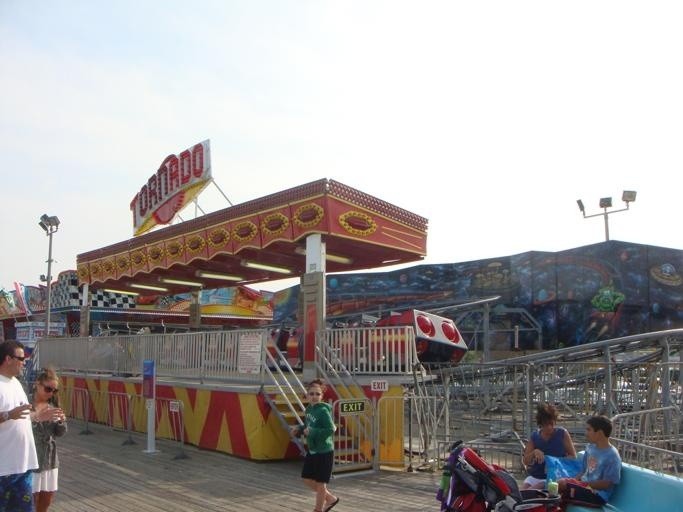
[548,482,559,497]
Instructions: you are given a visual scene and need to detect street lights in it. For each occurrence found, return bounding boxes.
[36,211,60,336]
[577,189,636,241]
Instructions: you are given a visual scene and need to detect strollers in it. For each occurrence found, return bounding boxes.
[437,440,561,512]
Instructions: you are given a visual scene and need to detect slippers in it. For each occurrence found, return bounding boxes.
[323,498,339,511]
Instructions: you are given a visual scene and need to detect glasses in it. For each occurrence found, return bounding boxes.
[14,357,24,361]
[41,384,58,394]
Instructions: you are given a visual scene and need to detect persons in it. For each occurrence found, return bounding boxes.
[290,378,339,512]
[518,400,576,490]
[25,366,68,512]
[0,339,40,512]
[556,415,621,512]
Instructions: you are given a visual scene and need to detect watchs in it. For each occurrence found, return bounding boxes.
[3,410,9,420]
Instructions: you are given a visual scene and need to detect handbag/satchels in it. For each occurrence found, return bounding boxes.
[544,455,584,489]
[450,491,486,512]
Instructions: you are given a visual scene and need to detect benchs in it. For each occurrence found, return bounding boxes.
[545,453,682,512]
